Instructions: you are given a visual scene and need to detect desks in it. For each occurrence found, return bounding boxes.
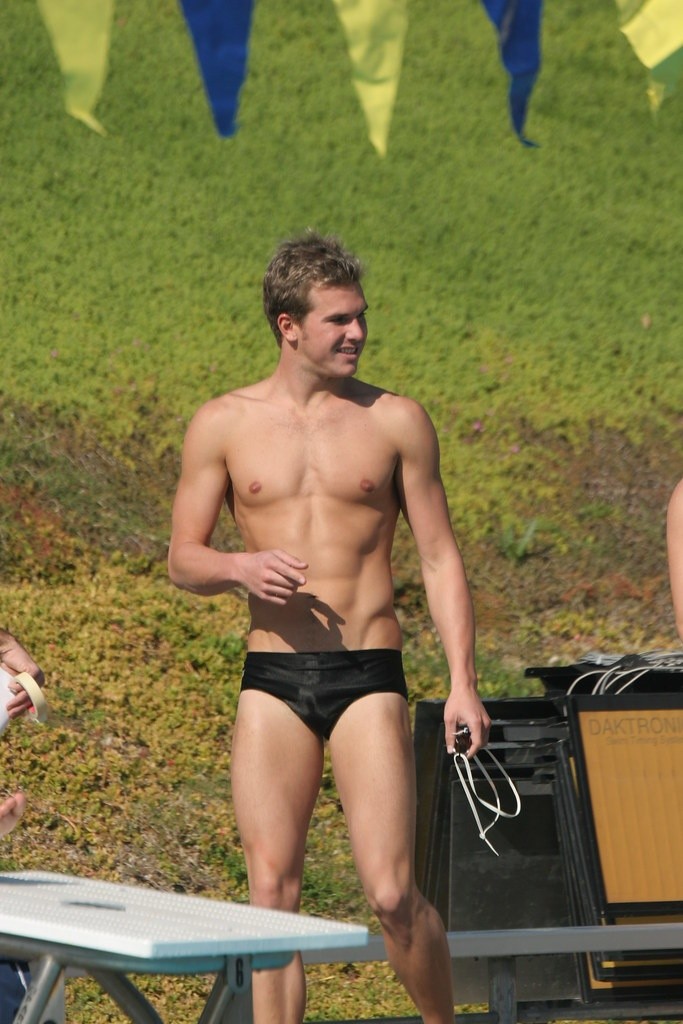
[0,867,370,1024]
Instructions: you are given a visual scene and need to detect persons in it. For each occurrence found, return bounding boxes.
[0,627,45,838]
[667,478,683,643]
[168,227,492,1024]
